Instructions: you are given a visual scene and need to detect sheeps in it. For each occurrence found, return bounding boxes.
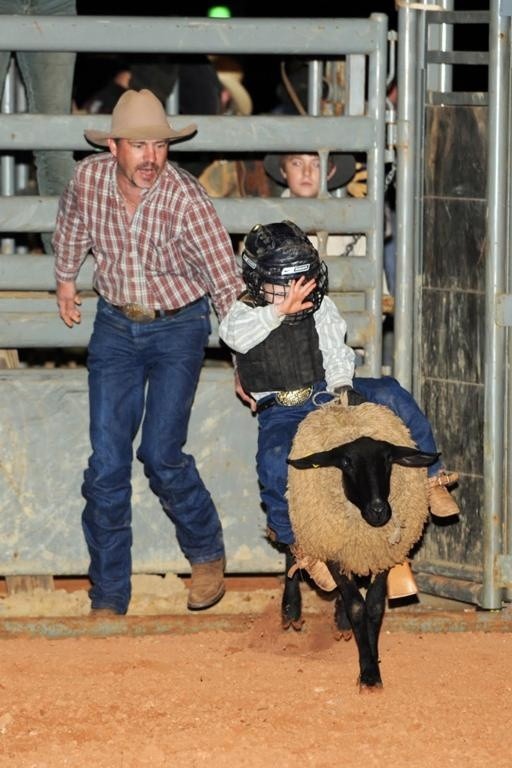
[262,400,441,694]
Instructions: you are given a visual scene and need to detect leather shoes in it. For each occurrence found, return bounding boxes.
[88,606,119,617]
[188,553,226,609]
[286,546,336,592]
[427,471,460,517]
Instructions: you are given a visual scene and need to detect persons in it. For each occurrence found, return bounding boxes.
[48,88,259,621]
[217,216,462,594]
[71,0,399,296]
[0,0,78,259]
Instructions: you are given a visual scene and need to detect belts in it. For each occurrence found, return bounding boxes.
[256,384,313,410]
[114,296,207,321]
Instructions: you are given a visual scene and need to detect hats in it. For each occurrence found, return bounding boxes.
[263,151,355,191]
[85,90,198,150]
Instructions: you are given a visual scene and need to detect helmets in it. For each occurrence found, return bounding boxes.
[242,221,329,324]
